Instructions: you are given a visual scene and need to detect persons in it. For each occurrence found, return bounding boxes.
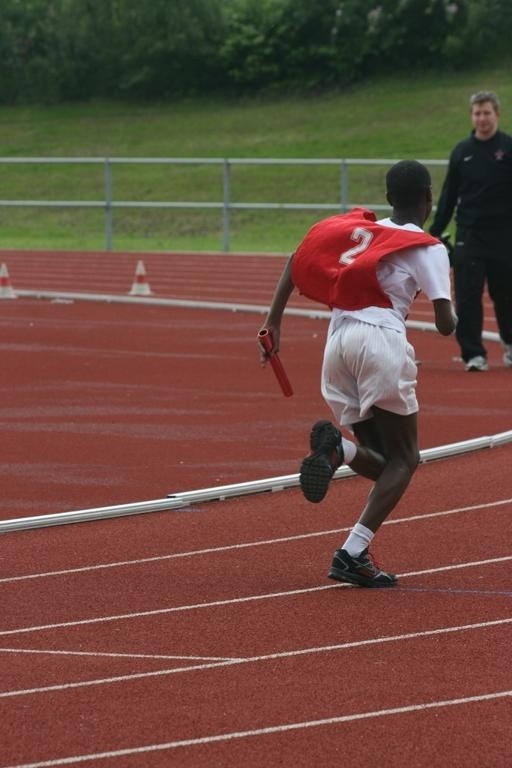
[257,160,460,587]
[429,90,512,372]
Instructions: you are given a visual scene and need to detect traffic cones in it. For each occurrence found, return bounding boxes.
[0,262,17,300]
[128,259,153,296]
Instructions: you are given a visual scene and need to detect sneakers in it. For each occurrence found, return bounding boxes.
[466,356,488,371]
[300,420,344,502]
[328,549,398,588]
[499,335,512,367]
